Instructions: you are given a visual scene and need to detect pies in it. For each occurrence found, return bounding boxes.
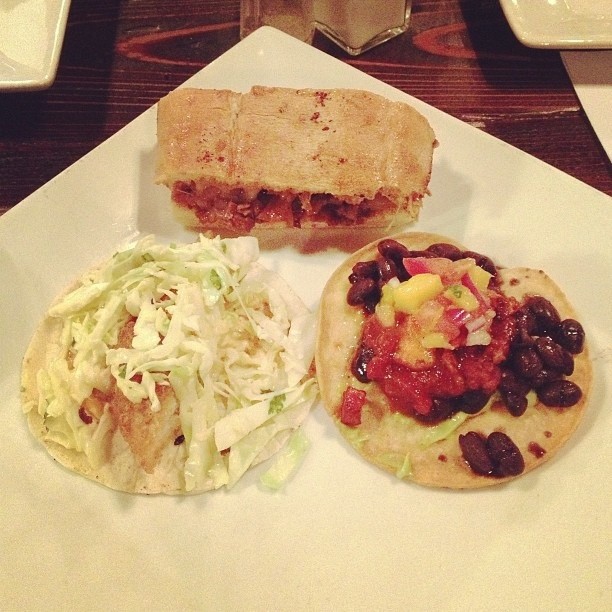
[316,233,594,491]
[22,234,316,497]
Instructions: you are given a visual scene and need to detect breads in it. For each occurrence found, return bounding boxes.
[155,82,435,231]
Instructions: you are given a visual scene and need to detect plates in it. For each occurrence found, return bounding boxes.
[500,1,612,50]
[1,23,612,610]
[1,2,73,92]
